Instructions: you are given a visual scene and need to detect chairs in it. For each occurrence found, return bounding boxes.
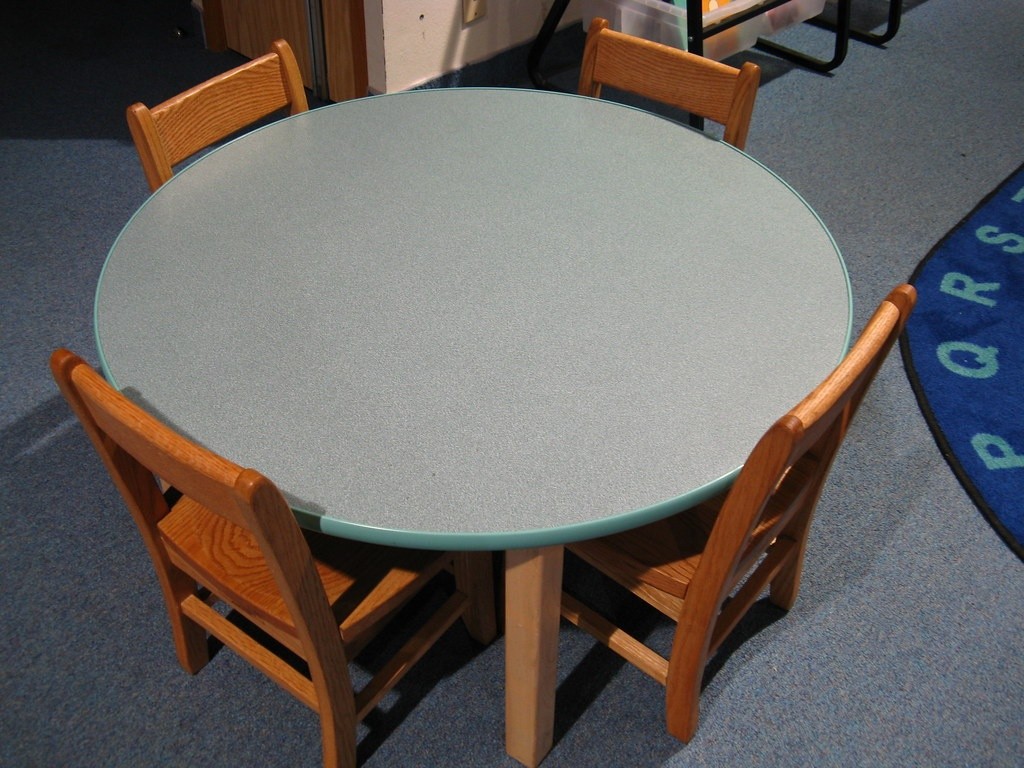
[43,346,486,768]
[570,14,767,149]
[561,275,925,743]
[121,37,310,193]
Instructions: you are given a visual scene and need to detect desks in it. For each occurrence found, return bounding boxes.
[87,85,856,768]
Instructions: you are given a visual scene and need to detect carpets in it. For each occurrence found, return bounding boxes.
[903,160,1024,560]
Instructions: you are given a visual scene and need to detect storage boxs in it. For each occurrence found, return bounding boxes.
[764,0,832,40]
[582,0,763,62]
